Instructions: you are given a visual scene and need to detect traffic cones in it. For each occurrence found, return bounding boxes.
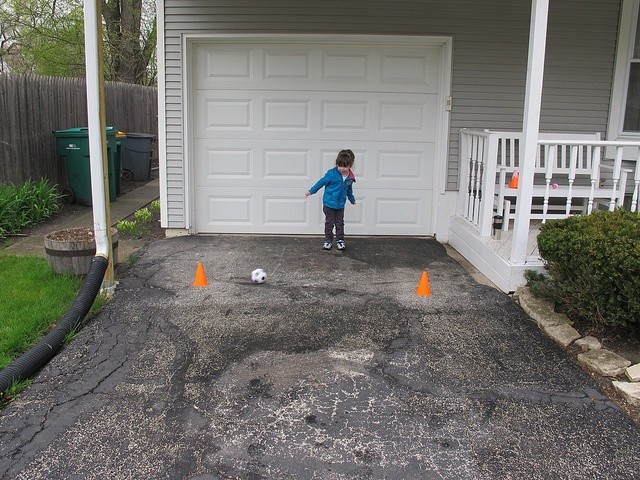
[416,271,432,296]
[193,263,208,286]
[509,168,519,188]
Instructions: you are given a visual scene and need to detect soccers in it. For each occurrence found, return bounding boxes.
[251,269,267,284]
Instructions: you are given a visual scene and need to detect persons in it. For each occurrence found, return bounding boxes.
[304,148,357,251]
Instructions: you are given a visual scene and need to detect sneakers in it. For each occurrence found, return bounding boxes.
[323,242,332,250]
[336,239,345,250]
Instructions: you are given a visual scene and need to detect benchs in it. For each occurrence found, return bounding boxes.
[484,128,635,231]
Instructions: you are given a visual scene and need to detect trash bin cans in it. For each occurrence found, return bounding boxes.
[121,132,156,182]
[53,128,118,207]
[117,131,126,197]
